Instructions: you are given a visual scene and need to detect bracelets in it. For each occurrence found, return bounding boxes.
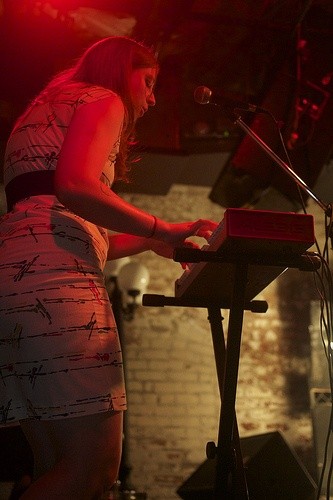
[148,216,156,236]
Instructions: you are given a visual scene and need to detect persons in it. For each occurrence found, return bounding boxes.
[0,36,218,500]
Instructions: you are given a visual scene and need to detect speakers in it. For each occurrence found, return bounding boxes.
[176,430,320,500]
[207,41,333,216]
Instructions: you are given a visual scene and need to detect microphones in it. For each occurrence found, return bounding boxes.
[193,86,269,114]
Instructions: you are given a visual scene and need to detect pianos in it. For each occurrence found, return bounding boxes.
[173,207,317,307]
